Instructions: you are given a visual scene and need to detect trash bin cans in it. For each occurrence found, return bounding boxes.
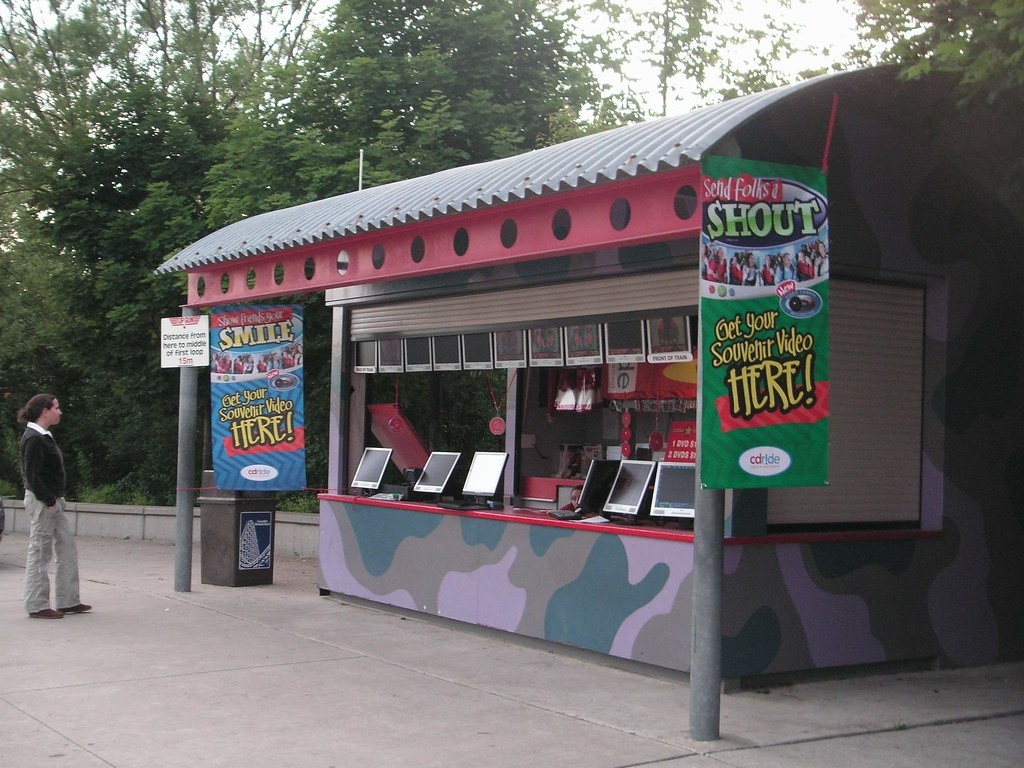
[196,469,275,587]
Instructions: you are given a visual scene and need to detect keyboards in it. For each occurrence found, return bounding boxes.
[547,509,582,520]
[439,500,483,510]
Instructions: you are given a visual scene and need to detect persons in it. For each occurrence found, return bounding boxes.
[762,253,799,285]
[18,394,93,619]
[729,251,760,288]
[560,485,584,511]
[211,343,303,373]
[701,242,727,283]
[797,241,830,279]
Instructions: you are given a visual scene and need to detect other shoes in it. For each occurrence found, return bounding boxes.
[30,609,63,619]
[59,604,92,614]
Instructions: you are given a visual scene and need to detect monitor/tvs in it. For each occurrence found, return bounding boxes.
[413,452,462,504]
[351,447,393,496]
[462,451,509,504]
[602,459,657,524]
[577,459,622,513]
[650,462,696,529]
